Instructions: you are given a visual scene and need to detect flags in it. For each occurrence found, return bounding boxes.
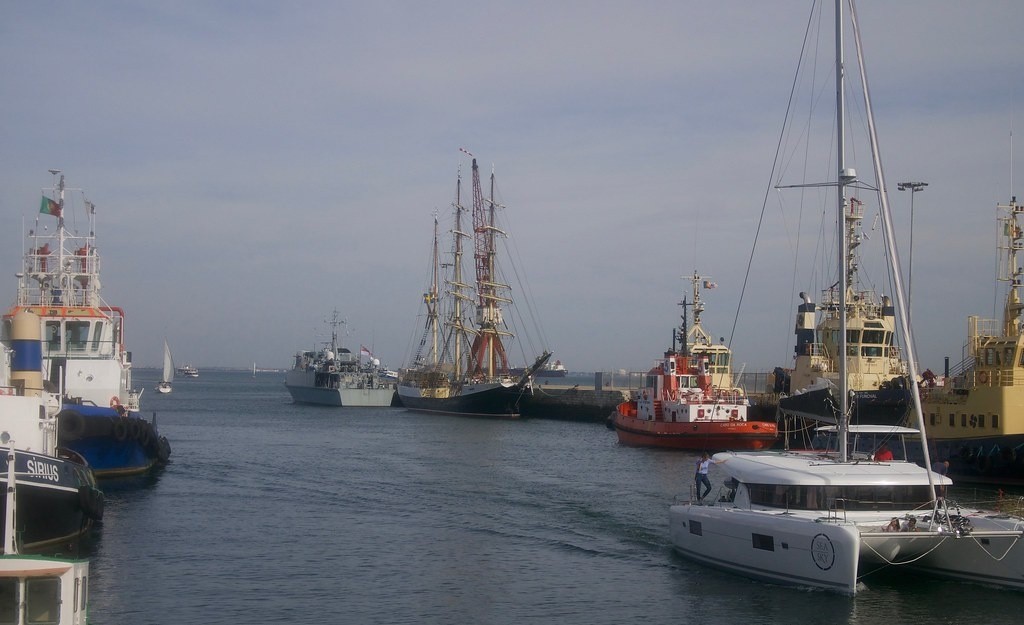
[359,345,371,356]
[40,195,61,217]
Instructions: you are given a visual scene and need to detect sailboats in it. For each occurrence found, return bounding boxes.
[157,338,175,393]
[665,0,1024,596]
[251,363,257,378]
[395,153,554,421]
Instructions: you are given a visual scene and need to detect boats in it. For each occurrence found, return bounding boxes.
[0,438,90,623]
[747,193,1023,474]
[284,308,395,409]
[176,367,199,379]
[1,305,103,550]
[475,359,568,379]
[1,166,168,488]
[609,268,778,452]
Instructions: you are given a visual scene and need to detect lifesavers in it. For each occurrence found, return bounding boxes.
[111,422,127,441]
[890,347,896,356]
[854,296,859,301]
[59,410,85,443]
[141,419,171,461]
[979,371,988,383]
[130,421,140,441]
[671,390,676,400]
[1000,446,1015,463]
[79,486,104,519]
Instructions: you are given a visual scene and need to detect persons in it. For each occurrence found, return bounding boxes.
[885,517,921,533]
[695,452,730,505]
[873,441,894,460]
[931,461,950,508]
